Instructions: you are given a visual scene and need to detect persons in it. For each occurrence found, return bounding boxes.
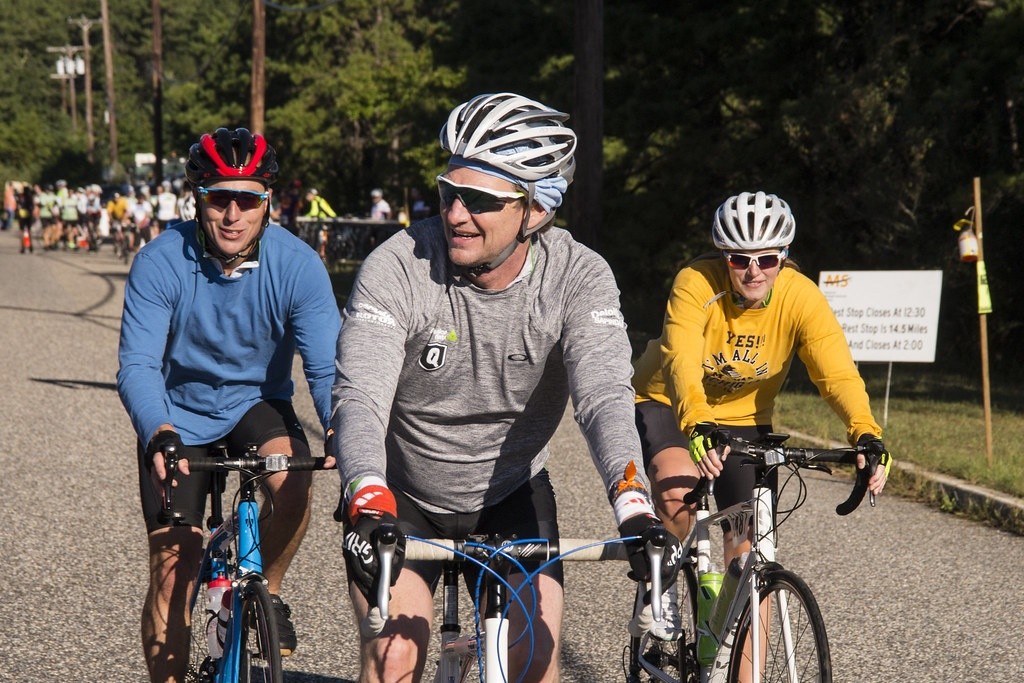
[0,175,304,255]
[397,201,410,227]
[410,185,430,219]
[330,93,682,683]
[629,192,893,683]
[299,185,336,262]
[116,128,343,683]
[368,188,392,221]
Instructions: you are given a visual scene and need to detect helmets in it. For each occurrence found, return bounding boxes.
[186,127,280,188]
[440,93,576,212]
[712,191,796,250]
[56,179,66,187]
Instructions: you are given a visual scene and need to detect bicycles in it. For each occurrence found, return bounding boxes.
[156,434,339,683]
[368,526,664,683]
[618,427,881,683]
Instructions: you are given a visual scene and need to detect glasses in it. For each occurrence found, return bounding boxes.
[723,250,785,269]
[437,173,524,214]
[197,186,268,209]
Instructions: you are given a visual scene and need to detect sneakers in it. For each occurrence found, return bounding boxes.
[637,572,682,640]
[250,594,296,656]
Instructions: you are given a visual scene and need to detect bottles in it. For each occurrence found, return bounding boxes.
[205,571,231,659]
[215,580,240,655]
[697,563,724,667]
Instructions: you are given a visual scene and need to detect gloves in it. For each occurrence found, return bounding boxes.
[618,514,683,604]
[343,508,406,607]
